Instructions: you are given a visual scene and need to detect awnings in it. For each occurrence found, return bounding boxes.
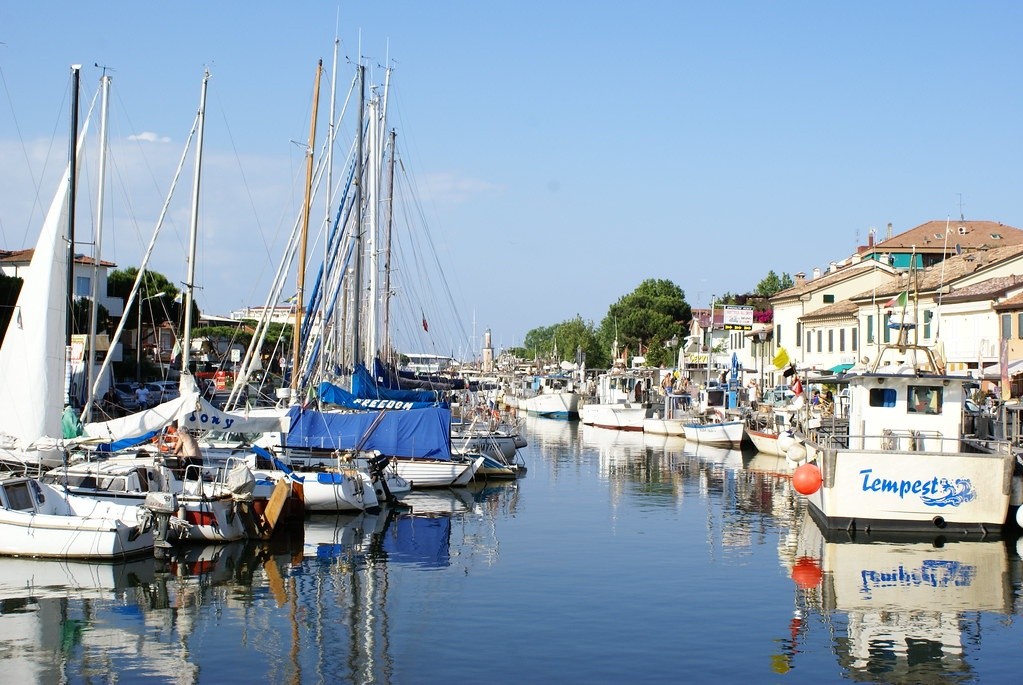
[801,374,857,384]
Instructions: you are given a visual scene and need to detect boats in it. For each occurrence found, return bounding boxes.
[783,505,1016,684]
[528,214,1014,535]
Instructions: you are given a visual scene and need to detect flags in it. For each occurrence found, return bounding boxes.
[884,291,907,308]
[783,365,797,378]
[773,348,790,370]
[423,313,429,332]
[789,378,803,395]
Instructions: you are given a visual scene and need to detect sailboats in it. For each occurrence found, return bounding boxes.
[679,294,745,449]
[0,7,527,589]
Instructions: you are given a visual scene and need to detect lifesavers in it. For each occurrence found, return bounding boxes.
[153,426,181,451]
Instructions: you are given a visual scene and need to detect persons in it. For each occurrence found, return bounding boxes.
[813,391,825,409]
[134,381,154,411]
[661,373,678,403]
[743,378,762,411]
[102,386,121,419]
[165,420,203,481]
[983,394,999,417]
[635,381,642,402]
[586,376,597,400]
[721,373,728,391]
[146,344,156,363]
[823,392,834,414]
